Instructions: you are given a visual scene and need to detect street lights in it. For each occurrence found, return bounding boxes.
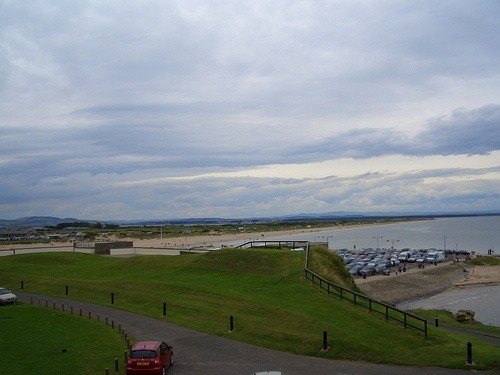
[372,236,384,247]
[321,236,333,248]
[386,239,400,249]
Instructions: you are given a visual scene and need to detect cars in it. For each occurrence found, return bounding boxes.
[334,246,450,276]
[0,287,17,305]
[2,231,111,240]
[125,341,175,374]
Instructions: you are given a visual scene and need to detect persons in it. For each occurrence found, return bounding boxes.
[394,262,409,275]
[362,271,368,283]
[416,258,439,270]
[448,248,495,264]
